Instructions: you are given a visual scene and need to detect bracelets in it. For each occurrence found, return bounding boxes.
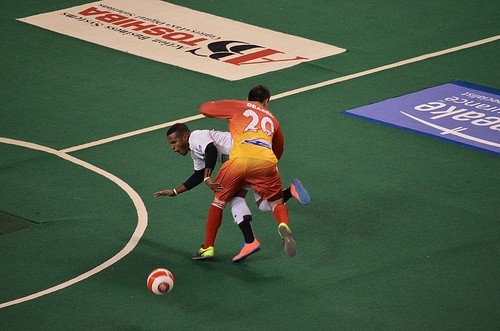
[203,176,211,183]
[173,188,177,196]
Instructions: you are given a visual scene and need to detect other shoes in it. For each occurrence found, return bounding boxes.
[232,238,261,262]
[277,222,297,257]
[192,244,215,259]
[290,178,311,205]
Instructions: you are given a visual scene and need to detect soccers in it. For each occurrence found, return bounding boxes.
[147,268,175,295]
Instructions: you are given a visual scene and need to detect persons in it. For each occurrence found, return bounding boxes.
[191,85,297,260]
[153,123,310,263]
